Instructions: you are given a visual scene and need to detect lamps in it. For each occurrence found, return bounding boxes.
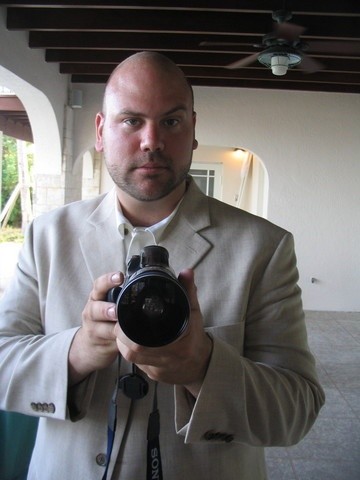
[254,9,312,77]
[232,146,249,159]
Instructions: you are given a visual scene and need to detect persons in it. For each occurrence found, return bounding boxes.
[0,51,325,480]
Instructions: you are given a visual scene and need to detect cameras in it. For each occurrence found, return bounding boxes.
[107,245,190,350]
